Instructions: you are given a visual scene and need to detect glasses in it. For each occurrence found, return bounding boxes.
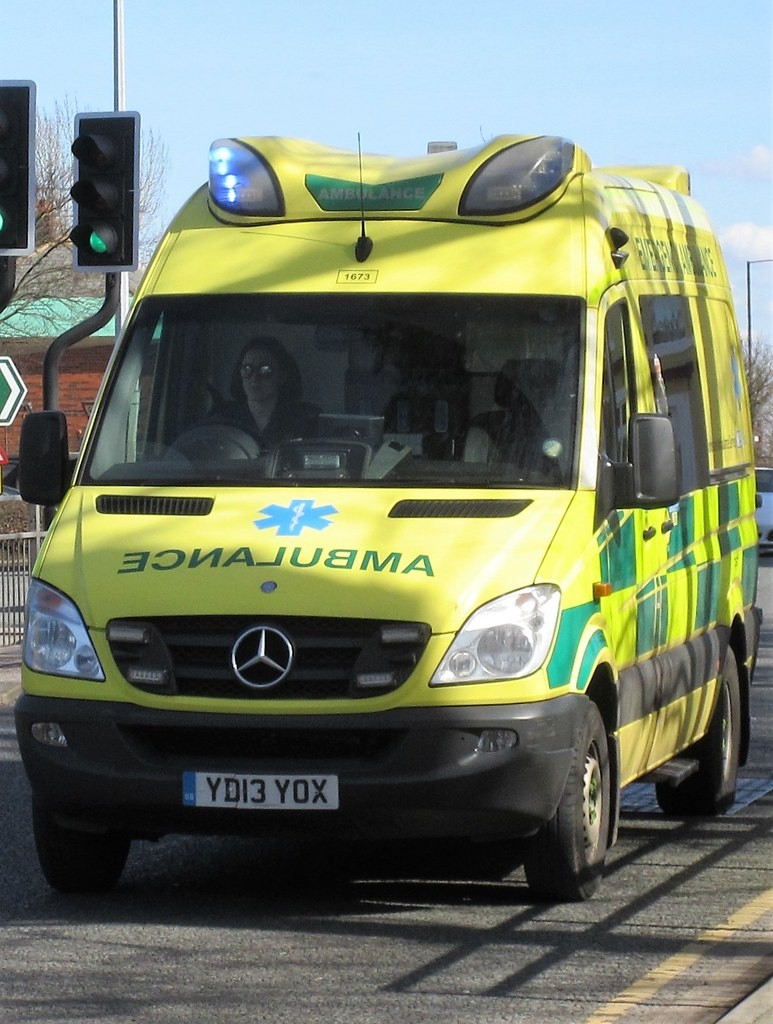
[238,362,279,380]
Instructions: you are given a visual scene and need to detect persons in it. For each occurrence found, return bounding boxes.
[197,340,321,446]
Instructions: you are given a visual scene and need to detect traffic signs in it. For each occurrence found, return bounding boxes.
[0,357,28,427]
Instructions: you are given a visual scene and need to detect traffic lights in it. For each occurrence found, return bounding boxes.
[69,109,138,272]
[0,80,37,258]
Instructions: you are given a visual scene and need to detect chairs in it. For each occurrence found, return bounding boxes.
[204,399,333,456]
[463,355,574,463]
